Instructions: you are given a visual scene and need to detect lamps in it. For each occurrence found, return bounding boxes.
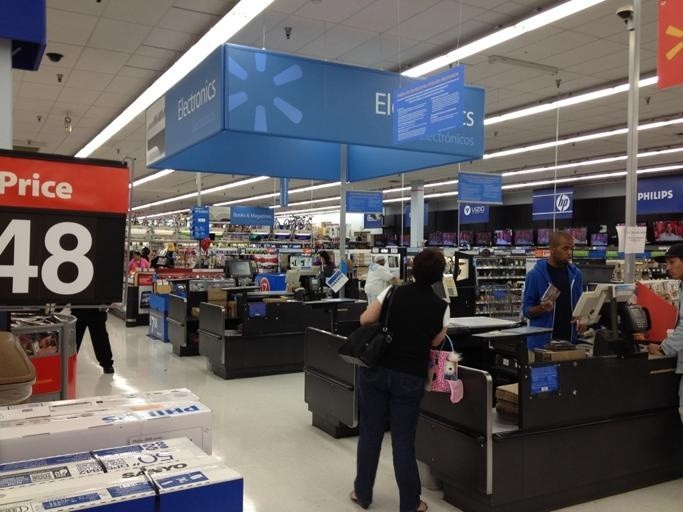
[69,0,681,221]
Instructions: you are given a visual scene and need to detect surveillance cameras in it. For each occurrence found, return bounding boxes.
[47,52,63,62]
[615,5,634,21]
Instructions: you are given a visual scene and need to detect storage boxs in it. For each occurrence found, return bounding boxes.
[0,385,246,512]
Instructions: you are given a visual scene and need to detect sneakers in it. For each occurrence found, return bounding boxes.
[103,365,114,374]
[413,499,428,512]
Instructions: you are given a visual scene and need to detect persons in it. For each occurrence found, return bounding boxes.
[347,248,451,512]
[140,247,149,263]
[151,250,156,257]
[481,248,490,257]
[523,229,586,365]
[658,222,677,241]
[57,300,115,374]
[128,251,150,272]
[363,254,398,308]
[648,244,682,433]
[316,251,335,288]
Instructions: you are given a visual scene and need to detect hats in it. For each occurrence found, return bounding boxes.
[653,243,683,263]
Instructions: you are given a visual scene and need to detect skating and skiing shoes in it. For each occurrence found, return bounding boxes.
[349,490,371,510]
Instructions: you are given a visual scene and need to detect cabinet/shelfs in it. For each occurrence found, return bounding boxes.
[473,255,527,320]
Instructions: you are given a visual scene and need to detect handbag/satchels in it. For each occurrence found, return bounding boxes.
[337,321,401,369]
[423,331,466,405]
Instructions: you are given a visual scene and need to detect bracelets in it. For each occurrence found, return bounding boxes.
[657,344,665,356]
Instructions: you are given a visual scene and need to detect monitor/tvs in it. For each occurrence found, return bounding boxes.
[227,261,253,278]
[413,274,451,303]
[574,282,635,329]
